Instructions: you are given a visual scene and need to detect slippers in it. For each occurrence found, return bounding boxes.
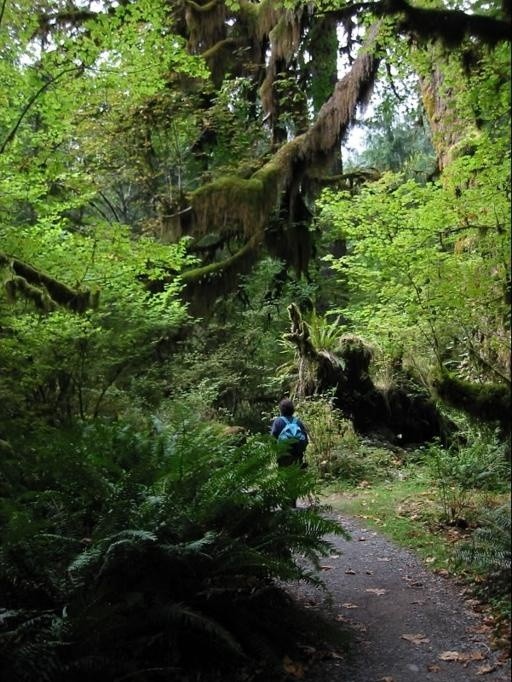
[277,416,306,443]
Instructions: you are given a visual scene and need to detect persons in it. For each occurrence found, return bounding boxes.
[269,399,309,513]
